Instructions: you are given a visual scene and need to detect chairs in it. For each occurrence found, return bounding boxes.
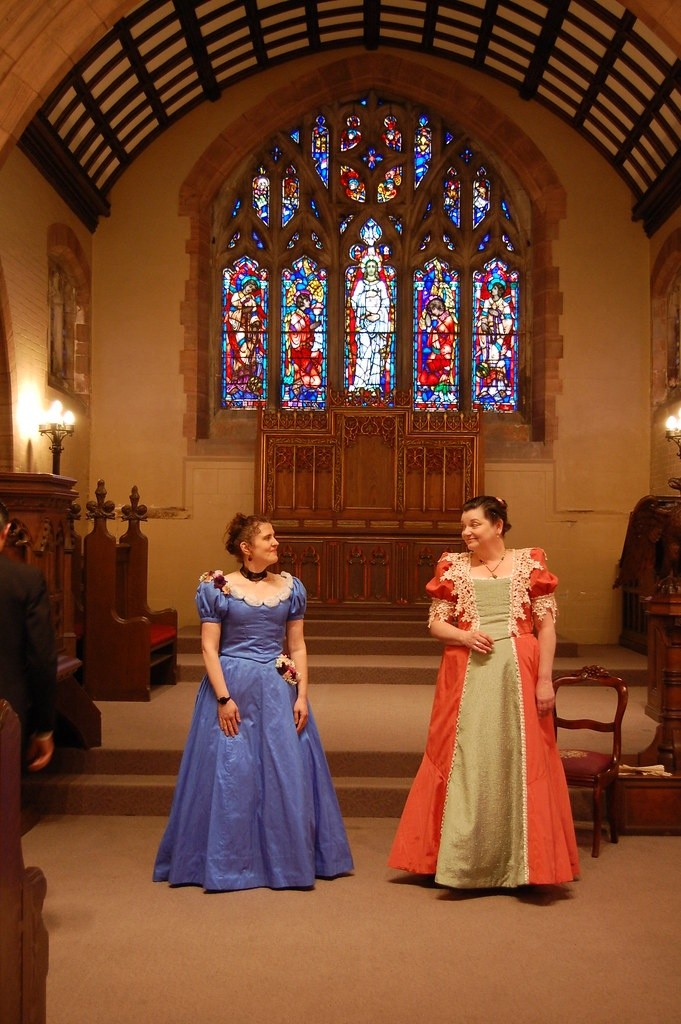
[550,664,628,858]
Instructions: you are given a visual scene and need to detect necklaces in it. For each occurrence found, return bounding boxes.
[479,549,506,579]
[239,566,267,582]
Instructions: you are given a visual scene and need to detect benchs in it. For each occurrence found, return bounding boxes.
[84,478,179,702]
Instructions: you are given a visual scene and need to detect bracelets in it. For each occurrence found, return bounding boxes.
[216,695,231,705]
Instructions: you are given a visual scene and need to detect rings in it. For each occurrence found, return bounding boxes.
[476,642,479,645]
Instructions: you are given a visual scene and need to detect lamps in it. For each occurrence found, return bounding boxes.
[38,399,77,475]
[664,408,681,492]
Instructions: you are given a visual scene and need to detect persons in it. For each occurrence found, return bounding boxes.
[152,512,355,890]
[0,503,57,773]
[388,495,583,890]
